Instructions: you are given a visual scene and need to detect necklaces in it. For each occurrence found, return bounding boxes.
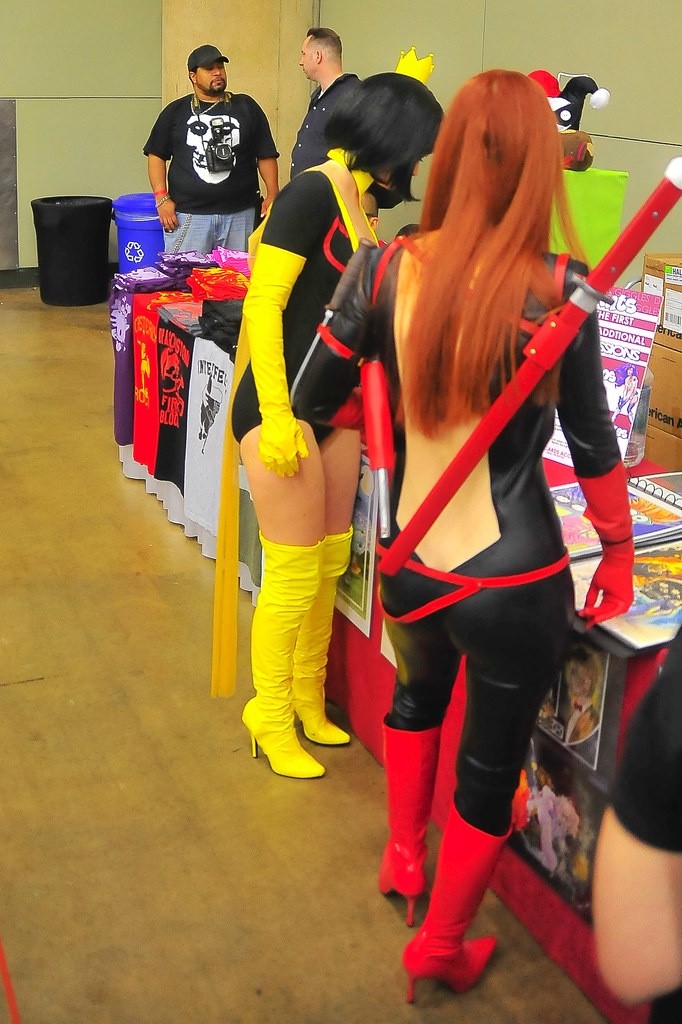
[190,97,223,115]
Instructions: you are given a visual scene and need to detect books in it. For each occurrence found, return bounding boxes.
[545,471,682,648]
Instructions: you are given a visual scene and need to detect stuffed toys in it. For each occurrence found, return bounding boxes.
[528,71,610,172]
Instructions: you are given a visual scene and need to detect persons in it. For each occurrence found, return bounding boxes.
[360,191,389,248]
[293,68,635,1002]
[230,73,444,780]
[592,620,682,1024]
[289,27,363,178]
[143,44,280,257]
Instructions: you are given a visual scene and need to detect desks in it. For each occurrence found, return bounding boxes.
[110,275,682,1024]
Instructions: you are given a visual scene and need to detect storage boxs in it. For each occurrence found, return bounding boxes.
[644,425,682,476]
[644,342,682,440]
[641,253,682,352]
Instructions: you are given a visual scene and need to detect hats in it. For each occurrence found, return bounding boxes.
[186,44,229,69]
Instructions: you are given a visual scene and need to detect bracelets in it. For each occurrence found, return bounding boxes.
[155,193,170,208]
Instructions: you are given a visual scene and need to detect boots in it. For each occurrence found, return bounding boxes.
[241,528,326,779]
[375,713,443,928]
[400,790,499,1006]
[293,527,351,745]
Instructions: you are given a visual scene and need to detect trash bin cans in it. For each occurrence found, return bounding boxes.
[112,193,164,275]
[30,196,113,307]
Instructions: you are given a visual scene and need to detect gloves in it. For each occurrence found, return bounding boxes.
[573,457,637,626]
[325,392,367,432]
[240,241,310,478]
